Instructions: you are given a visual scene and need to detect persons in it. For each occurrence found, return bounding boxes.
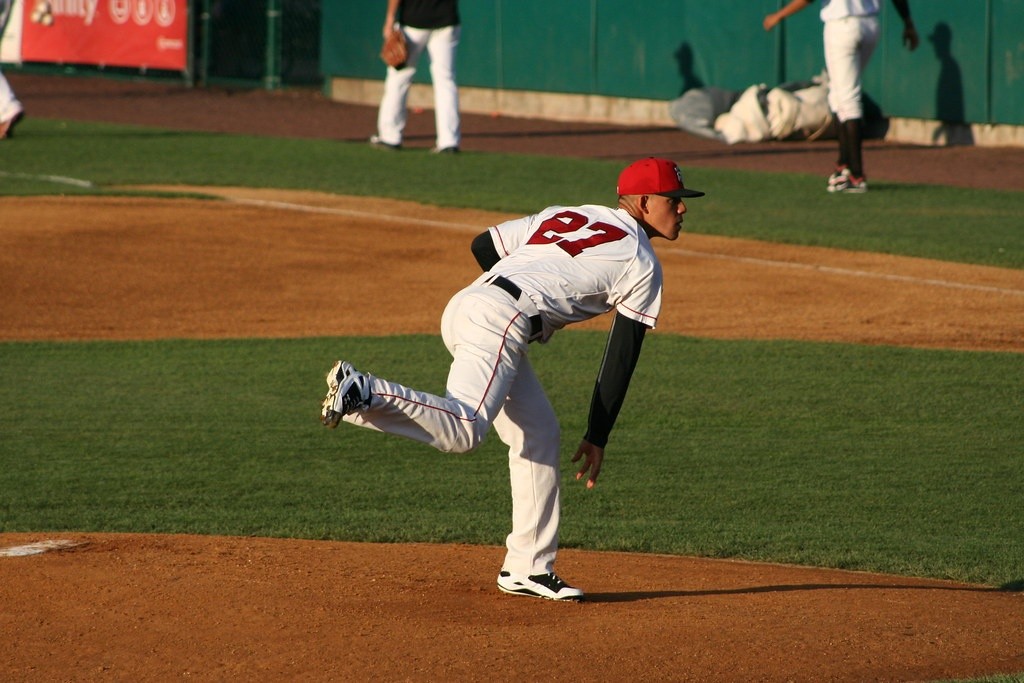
[321,156,706,601]
[369,0,462,152]
[0,0,25,139]
[764,0,920,193]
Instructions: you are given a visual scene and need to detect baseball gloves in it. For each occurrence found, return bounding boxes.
[381,29,411,69]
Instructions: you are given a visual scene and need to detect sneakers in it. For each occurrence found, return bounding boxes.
[827,165,866,193]
[321,360,372,429]
[496,567,584,602]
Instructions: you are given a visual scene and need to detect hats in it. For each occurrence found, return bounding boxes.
[617,157,705,198]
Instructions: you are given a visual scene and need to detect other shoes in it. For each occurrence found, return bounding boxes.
[370,135,402,152]
[432,147,459,154]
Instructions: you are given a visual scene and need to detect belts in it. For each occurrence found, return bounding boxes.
[486,275,542,344]
